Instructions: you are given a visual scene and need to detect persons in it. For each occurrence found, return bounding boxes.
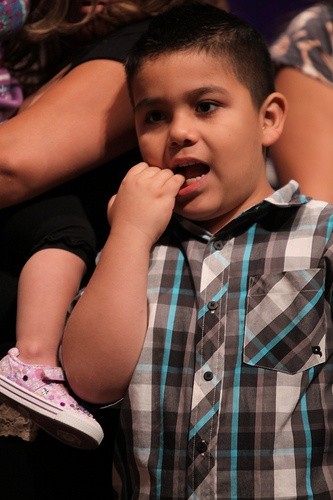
[1,0,104,443]
[58,0,333,500]
[260,0,331,202]
[1,0,186,500]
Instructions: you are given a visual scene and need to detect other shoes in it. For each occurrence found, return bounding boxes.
[0,350,105,448]
